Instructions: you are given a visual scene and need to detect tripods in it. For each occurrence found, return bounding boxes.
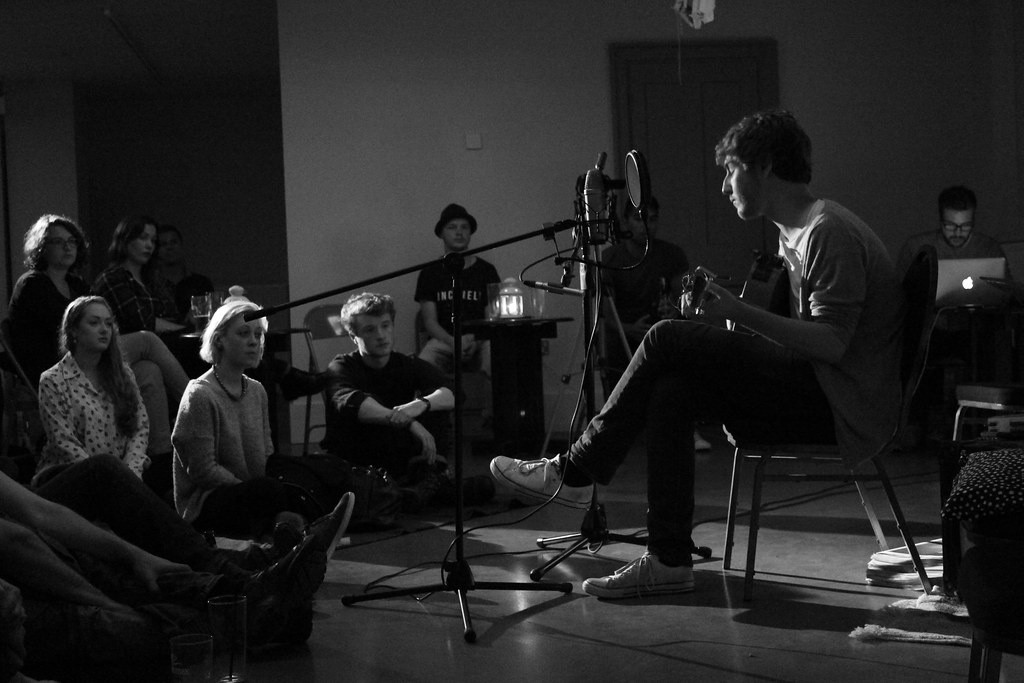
[243,215,713,639]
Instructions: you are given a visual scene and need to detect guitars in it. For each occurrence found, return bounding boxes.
[676,249,790,341]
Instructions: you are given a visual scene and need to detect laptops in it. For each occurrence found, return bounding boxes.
[934,256,1006,305]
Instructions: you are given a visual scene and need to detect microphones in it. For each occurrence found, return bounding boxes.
[523,280,585,298]
[584,169,609,242]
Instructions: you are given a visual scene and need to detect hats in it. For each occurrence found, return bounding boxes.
[434,203,478,237]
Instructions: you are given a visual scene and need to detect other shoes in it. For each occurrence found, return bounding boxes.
[400,488,424,515]
[897,425,918,452]
[456,475,494,505]
[694,431,712,450]
[301,491,356,562]
[241,535,319,649]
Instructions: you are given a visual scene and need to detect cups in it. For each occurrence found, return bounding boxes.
[191,295,212,332]
[207,595,246,683]
[169,634,213,683]
[206,291,226,319]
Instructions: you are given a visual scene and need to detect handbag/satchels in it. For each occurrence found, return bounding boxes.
[338,465,402,531]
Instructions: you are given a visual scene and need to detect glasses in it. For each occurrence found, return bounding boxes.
[941,219,973,232]
[48,236,80,248]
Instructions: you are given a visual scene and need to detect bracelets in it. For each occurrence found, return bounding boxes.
[415,396,431,413]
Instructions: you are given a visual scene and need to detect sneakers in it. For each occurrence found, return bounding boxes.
[490,455,593,511]
[583,551,695,598]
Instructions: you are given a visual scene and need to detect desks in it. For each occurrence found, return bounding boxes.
[465,317,574,452]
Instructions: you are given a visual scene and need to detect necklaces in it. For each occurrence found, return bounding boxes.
[211,364,248,401]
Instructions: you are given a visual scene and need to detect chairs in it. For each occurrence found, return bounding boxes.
[721,304,944,601]
[302,304,352,456]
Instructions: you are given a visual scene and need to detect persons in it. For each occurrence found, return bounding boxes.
[317,289,468,512]
[601,194,713,451]
[87,213,192,499]
[490,111,904,597]
[0,466,331,653]
[140,224,215,331]
[30,295,307,594]
[890,184,1014,446]
[31,454,355,598]
[416,203,501,380]
[0,211,92,457]
[170,296,333,540]
